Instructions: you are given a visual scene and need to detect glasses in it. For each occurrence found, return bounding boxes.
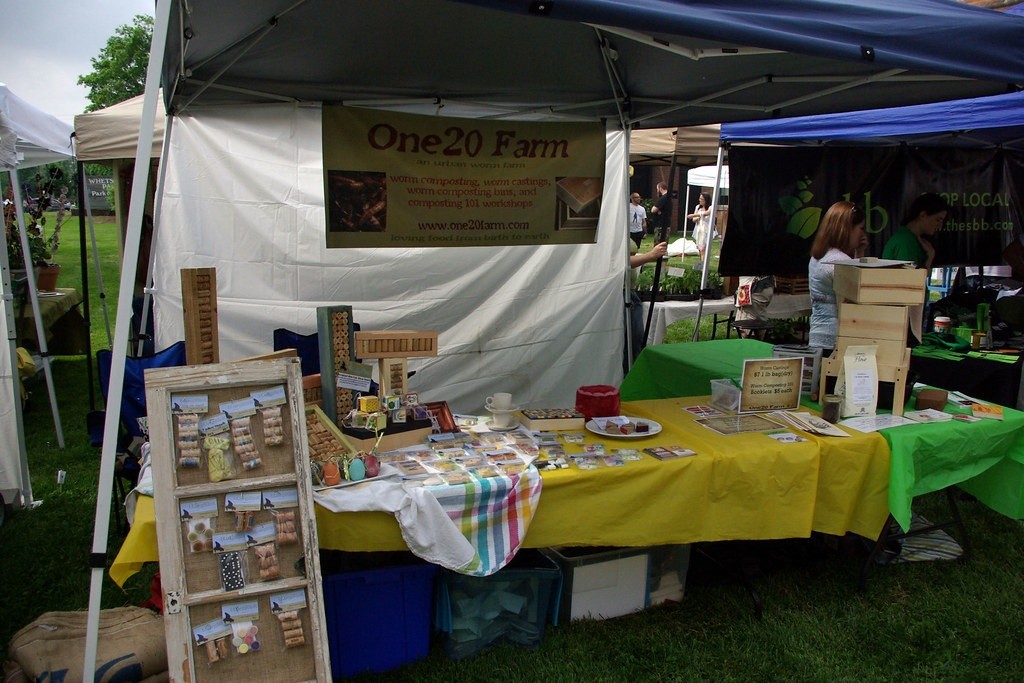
[851,204,862,213]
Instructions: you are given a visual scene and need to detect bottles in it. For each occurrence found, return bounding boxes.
[822,394,842,424]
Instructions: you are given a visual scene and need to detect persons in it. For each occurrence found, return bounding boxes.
[734,275,777,340]
[630,242,666,269]
[688,193,712,262]
[651,182,671,247]
[883,192,948,277]
[629,192,647,248]
[809,201,869,358]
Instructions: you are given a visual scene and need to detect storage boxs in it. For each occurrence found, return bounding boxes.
[441,546,560,646]
[319,549,436,680]
[543,546,652,623]
[10,267,27,299]
[36,264,60,290]
[831,261,926,366]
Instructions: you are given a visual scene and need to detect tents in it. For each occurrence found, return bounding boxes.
[0,80,113,508]
[682,166,729,262]
[74,87,165,411]
[81,2,1024,683]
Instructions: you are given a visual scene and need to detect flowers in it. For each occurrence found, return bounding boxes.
[1,163,77,274]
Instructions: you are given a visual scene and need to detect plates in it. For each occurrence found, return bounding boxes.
[585,417,663,439]
[485,421,519,430]
[484,404,520,413]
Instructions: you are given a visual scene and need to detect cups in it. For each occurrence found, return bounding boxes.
[493,414,511,427]
[486,392,512,410]
[933,316,951,334]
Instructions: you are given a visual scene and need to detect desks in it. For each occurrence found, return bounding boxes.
[12,285,84,349]
[617,339,1022,596]
[106,391,893,602]
[640,293,812,346]
